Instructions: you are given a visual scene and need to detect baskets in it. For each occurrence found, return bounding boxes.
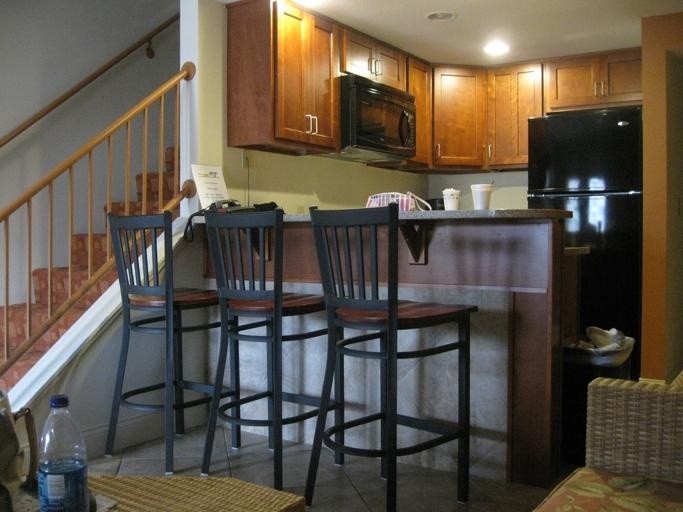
[364,192,415,212]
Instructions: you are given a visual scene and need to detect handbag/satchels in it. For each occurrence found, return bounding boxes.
[0,390,24,511]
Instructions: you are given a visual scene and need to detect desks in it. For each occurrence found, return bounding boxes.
[0,477,306,512]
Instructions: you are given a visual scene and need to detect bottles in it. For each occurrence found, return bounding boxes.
[36,394,92,512]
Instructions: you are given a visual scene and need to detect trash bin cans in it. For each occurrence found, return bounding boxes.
[562,334,636,467]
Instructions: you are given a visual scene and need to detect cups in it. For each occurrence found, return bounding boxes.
[471,183,494,210]
[441,189,462,211]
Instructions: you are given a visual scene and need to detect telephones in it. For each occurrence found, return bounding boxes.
[209,199,256,213]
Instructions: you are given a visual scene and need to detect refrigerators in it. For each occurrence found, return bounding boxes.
[525,103,645,475]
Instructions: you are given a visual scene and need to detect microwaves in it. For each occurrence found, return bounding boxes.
[316,72,417,164]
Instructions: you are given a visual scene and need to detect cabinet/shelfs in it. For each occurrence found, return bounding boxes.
[434,64,485,173]
[226,0,340,157]
[407,52,434,171]
[341,24,408,93]
[484,59,544,172]
[543,46,642,114]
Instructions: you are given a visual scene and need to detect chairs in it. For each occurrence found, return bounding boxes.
[303,203,478,512]
[531,376,683,512]
[200,207,343,490]
[103,209,239,473]
